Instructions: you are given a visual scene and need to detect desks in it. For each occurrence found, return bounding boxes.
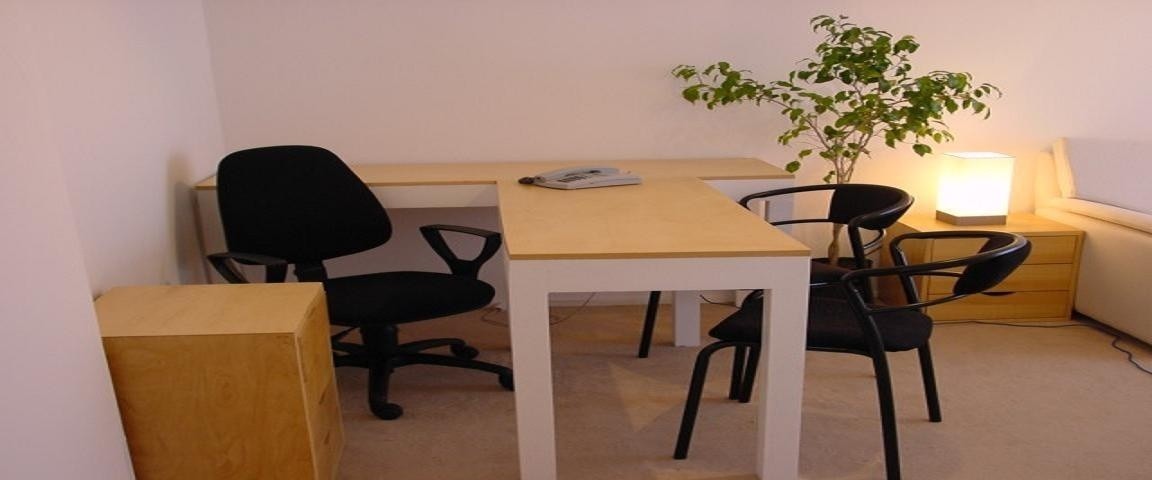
[181,153,803,480]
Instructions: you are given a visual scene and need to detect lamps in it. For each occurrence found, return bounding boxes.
[935,151,1016,228]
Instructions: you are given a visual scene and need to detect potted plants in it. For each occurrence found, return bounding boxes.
[670,14,1004,314]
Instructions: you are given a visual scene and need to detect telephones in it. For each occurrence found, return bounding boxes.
[531,166,642,190]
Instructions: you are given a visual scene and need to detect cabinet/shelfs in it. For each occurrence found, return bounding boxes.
[886,217,1086,320]
[94,283,346,480]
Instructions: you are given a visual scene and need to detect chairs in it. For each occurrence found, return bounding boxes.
[671,233,1031,476]
[206,135,511,419]
[626,183,916,398]
